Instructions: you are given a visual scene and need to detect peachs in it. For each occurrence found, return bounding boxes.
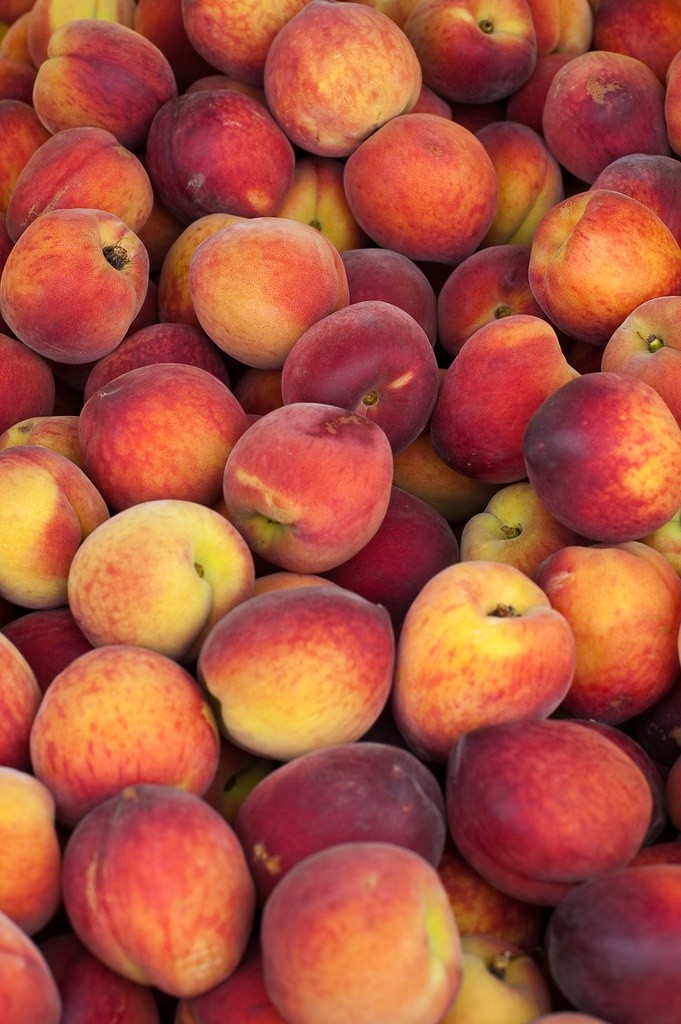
[0,0,681,1024]
